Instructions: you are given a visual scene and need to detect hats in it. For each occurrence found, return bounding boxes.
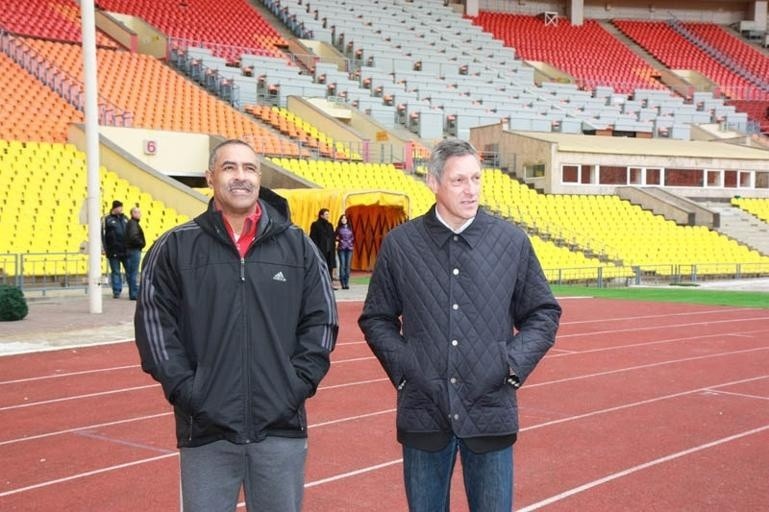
[111,199,123,209]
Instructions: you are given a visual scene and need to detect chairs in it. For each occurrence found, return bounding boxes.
[0,1,769,285]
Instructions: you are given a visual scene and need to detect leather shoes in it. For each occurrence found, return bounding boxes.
[114,293,118,298]
[129,296,137,300]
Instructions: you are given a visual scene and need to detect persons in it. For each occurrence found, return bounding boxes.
[356,138,563,512]
[134,139,340,512]
[102,201,128,298]
[335,215,355,289]
[309,209,337,290]
[125,207,146,300]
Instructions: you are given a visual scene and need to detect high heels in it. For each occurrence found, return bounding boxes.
[341,284,349,290]
